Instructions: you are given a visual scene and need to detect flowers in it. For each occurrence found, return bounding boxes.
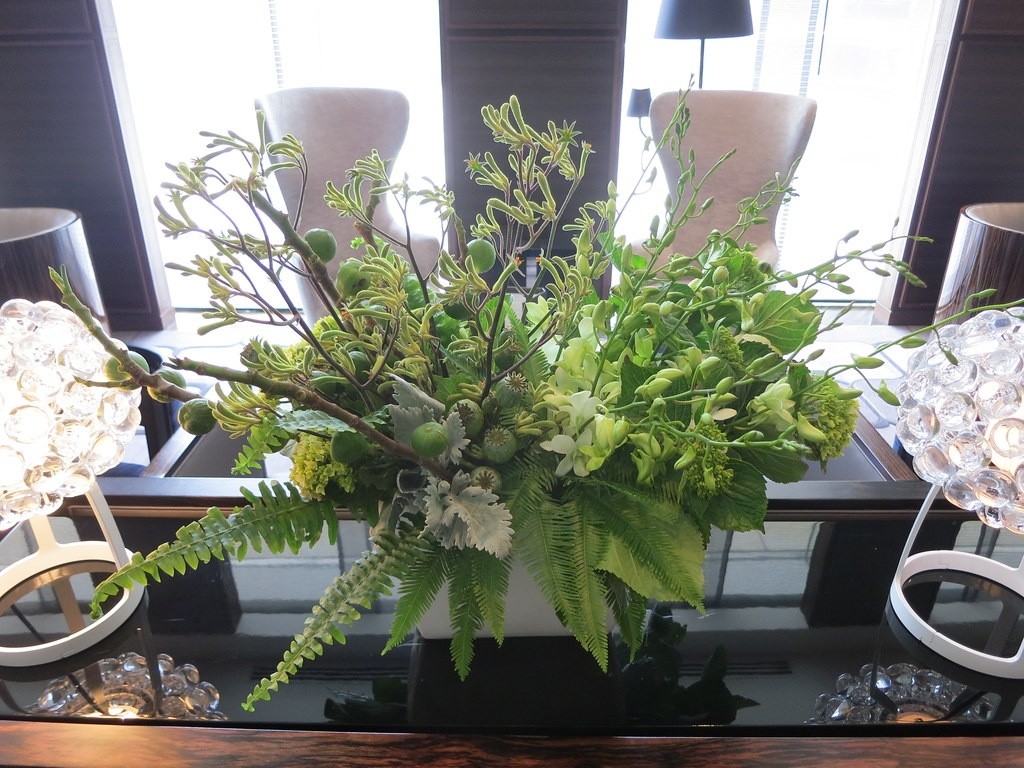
[41,60,995,715]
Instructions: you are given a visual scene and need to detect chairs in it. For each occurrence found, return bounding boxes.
[254,86,441,343]
[618,89,819,301]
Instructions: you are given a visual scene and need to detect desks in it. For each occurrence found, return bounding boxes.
[0,339,1024,768]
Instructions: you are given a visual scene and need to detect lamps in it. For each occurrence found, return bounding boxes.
[15,650,228,722]
[625,88,655,141]
[885,200,1024,695]
[653,0,754,90]
[0,207,143,686]
[802,660,1009,723]
